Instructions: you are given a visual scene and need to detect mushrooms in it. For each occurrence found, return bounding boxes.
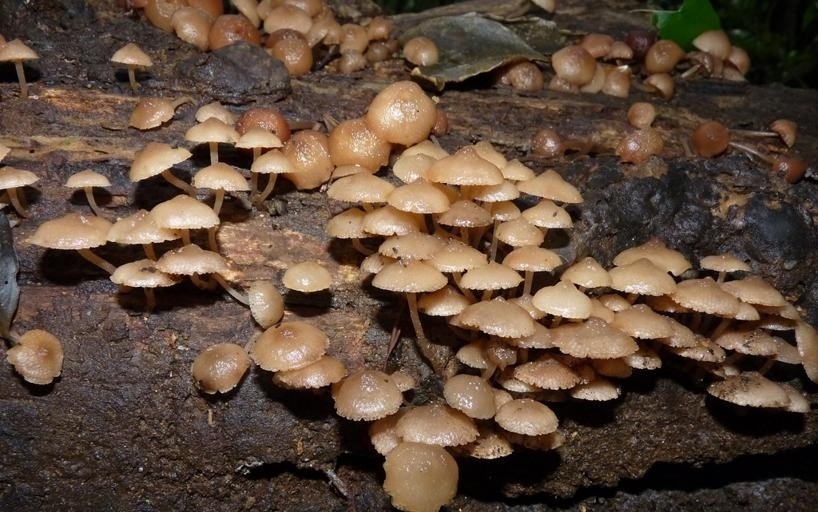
[0,0,817,512]
